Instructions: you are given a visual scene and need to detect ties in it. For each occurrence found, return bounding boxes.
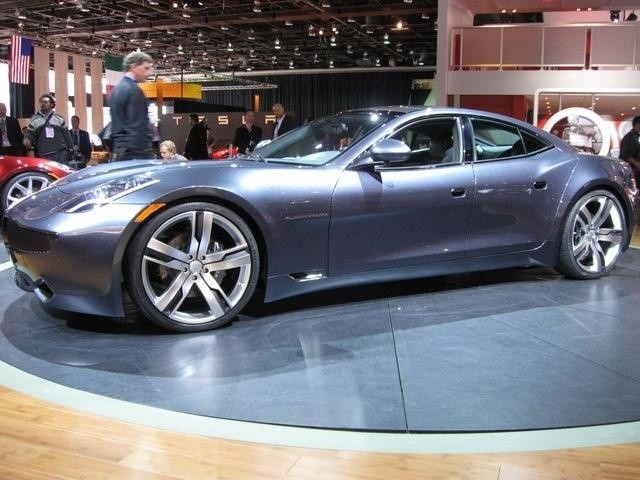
[0,118,6,145]
[73,131,79,146]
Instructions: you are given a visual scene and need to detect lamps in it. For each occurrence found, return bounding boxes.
[16,0,439,70]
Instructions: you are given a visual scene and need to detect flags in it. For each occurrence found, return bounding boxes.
[8,36,33,86]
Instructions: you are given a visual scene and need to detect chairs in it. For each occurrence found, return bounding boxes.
[428,134,454,162]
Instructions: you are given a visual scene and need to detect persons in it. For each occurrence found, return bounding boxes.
[184,113,209,160]
[271,103,295,139]
[20,126,28,137]
[20,94,77,164]
[200,117,211,142]
[67,116,92,170]
[233,110,263,153]
[618,114,639,189]
[158,139,189,160]
[109,50,157,160]
[0,102,28,159]
